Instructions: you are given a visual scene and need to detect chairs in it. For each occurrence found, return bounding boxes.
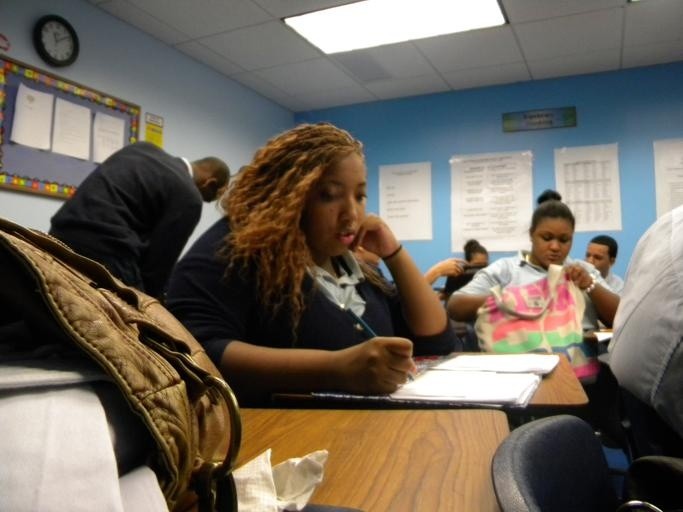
[491,361,683,512]
[450,318,478,350]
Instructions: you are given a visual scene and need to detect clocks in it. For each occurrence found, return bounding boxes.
[31,13,80,68]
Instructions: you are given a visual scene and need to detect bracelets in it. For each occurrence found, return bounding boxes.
[585,274,596,293]
[382,243,403,261]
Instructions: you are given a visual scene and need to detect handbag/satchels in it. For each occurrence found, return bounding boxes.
[473,263,600,389]
[0,211,244,511]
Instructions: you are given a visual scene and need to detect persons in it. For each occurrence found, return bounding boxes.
[164,122,456,405]
[354,246,470,287]
[48,141,230,293]
[597,205,683,451]
[464,240,489,266]
[446,189,620,393]
[585,236,625,328]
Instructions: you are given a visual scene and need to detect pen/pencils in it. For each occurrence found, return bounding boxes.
[346,308,414,380]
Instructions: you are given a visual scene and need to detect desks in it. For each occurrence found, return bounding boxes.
[224,406,513,511]
[583,328,613,361]
[278,351,589,410]
